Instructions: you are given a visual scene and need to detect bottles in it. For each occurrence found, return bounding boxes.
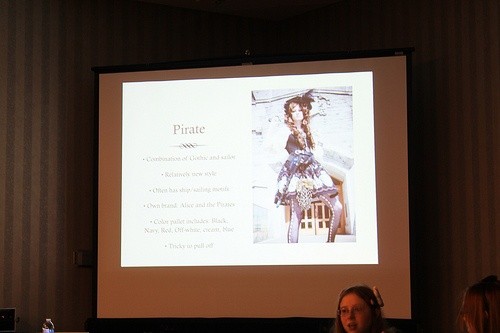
[42,318,54,333]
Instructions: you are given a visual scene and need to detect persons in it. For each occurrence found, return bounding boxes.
[273,97,344,243]
[333,285,391,333]
[463,275,500,333]
